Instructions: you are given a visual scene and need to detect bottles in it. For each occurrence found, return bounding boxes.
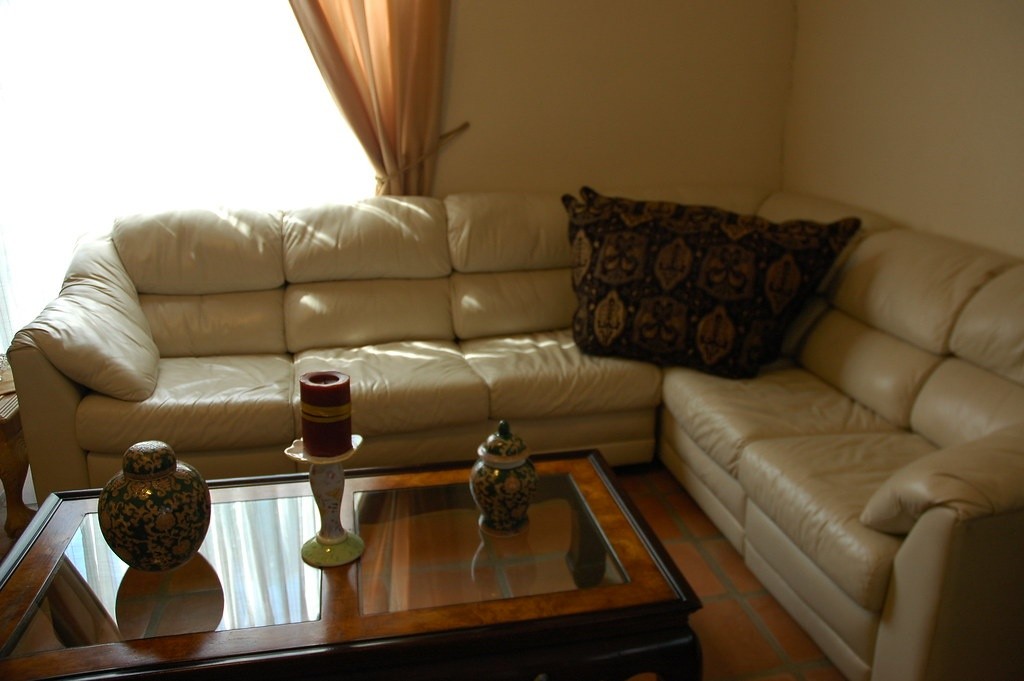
[98,440,212,575]
[470,418,536,540]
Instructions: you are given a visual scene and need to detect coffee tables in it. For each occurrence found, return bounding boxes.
[0,447,706,681]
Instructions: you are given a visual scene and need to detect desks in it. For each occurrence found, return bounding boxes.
[0,350,41,541]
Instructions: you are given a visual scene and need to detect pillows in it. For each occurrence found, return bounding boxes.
[560,193,833,379]
[579,185,863,360]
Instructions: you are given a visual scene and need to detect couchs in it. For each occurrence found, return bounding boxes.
[7,191,1024,681]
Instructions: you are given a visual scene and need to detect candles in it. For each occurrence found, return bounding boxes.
[299,371,352,458]
